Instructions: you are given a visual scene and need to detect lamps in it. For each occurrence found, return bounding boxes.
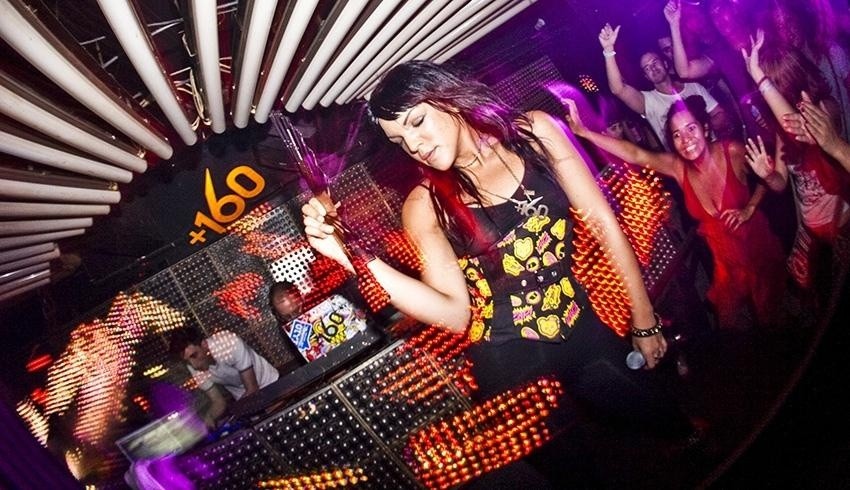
[0,0,540,307]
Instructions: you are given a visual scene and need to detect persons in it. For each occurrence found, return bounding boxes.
[168,324,282,432]
[560,1,850,391]
[300,62,707,489]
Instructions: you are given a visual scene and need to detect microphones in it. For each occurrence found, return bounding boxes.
[626,334,681,370]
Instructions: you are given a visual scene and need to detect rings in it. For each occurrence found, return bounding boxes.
[654,352,660,358]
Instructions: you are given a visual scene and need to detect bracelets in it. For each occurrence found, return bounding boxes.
[628,312,663,339]
